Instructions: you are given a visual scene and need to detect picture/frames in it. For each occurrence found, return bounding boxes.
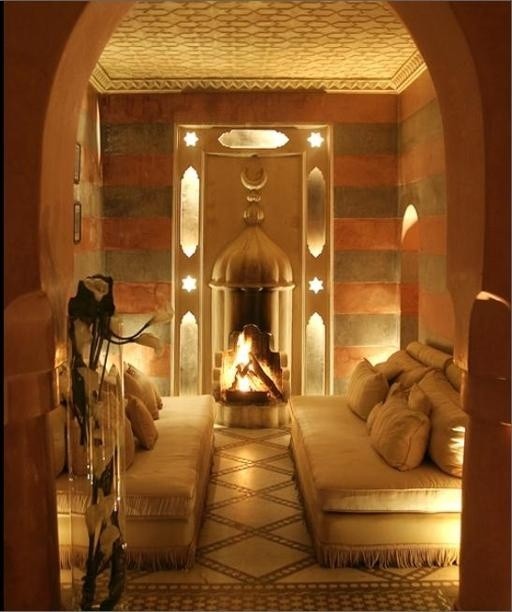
[73,203,81,244]
[74,142,82,185]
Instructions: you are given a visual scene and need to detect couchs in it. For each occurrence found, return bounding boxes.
[68,394,216,570]
[287,341,469,566]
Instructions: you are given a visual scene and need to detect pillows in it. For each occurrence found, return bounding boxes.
[123,361,161,450]
[344,349,467,478]
